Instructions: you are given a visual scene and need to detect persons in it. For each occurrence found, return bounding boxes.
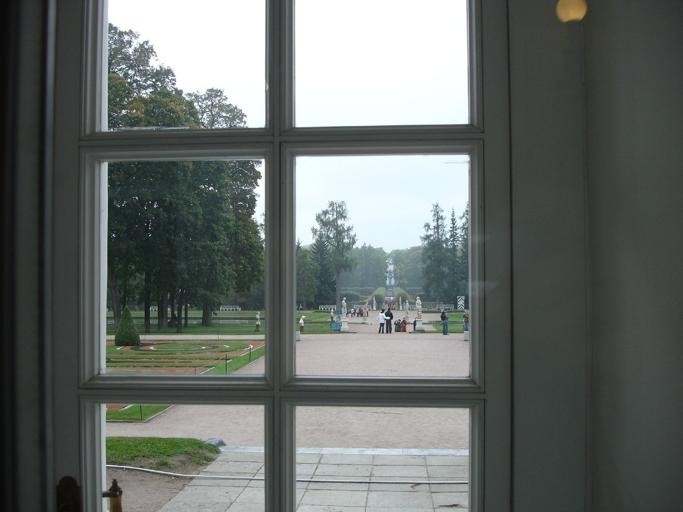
[393,318,401,332]
[341,296,348,318]
[440,308,448,336]
[462,310,469,331]
[416,296,423,320]
[384,255,398,285]
[298,314,305,331]
[405,300,410,315]
[254,310,262,333]
[349,303,368,318]
[399,316,407,332]
[376,308,391,334]
[384,307,393,333]
